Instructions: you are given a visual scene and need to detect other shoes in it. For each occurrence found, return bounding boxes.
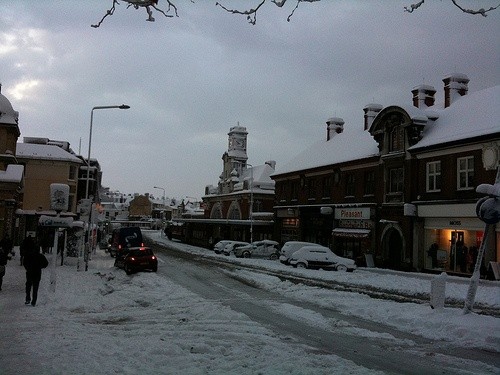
[25,300,30,304]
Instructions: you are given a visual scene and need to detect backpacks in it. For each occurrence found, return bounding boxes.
[37,253,48,268]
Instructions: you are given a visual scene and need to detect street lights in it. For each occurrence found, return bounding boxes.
[153,186,166,214]
[230,161,254,244]
[79,104,130,257]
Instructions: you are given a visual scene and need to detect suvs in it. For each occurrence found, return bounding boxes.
[279,242,323,266]
[213,240,249,256]
[234,239,280,261]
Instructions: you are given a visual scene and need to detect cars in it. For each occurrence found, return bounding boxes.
[99,233,112,249]
[289,246,357,273]
[114,246,158,275]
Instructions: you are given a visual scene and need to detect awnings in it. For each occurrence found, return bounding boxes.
[332,228,372,241]
[39,216,85,229]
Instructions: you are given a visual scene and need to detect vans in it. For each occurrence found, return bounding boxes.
[108,225,144,258]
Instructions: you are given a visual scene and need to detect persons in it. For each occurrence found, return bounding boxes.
[19,235,65,265]
[428,241,486,273]
[0,234,15,291]
[25,246,48,306]
